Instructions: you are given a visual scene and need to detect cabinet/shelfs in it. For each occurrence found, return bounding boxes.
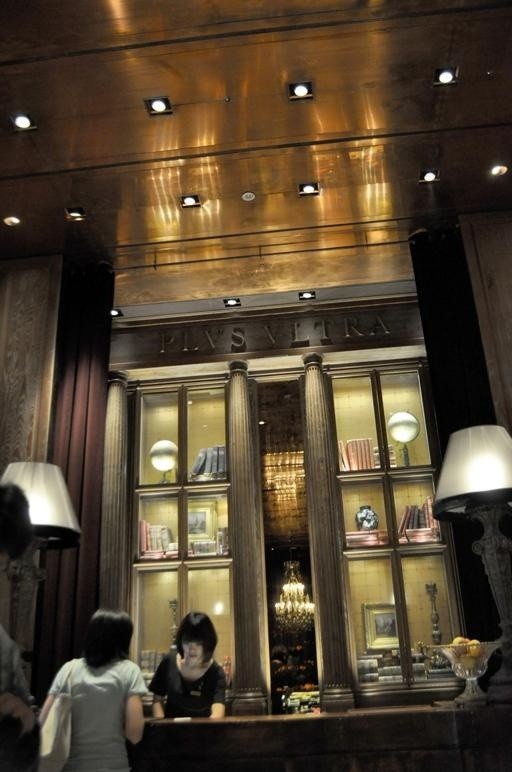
[328,361,468,696]
[127,377,236,705]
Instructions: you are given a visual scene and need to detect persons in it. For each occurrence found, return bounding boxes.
[149,611,226,720]
[37,605,146,772]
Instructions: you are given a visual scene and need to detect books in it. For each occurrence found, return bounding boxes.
[378,665,402,675]
[190,448,207,475]
[216,527,229,554]
[347,438,375,471]
[219,447,226,473]
[190,539,217,554]
[397,496,441,544]
[357,659,378,675]
[151,526,172,550]
[141,650,167,706]
[204,448,212,473]
[377,675,403,683]
[212,447,218,473]
[169,543,179,551]
[373,445,397,469]
[358,673,378,683]
[338,440,351,471]
[412,663,425,673]
[140,520,146,552]
[414,674,427,681]
[146,523,151,552]
[427,669,458,679]
[356,654,382,659]
[345,529,388,548]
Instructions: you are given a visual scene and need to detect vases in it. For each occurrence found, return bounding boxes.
[354,505,380,531]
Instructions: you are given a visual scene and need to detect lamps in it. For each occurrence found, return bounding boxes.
[0,461,83,700]
[433,425,511,703]
[275,547,315,635]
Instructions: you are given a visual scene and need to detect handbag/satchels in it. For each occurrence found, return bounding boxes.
[38,658,76,772]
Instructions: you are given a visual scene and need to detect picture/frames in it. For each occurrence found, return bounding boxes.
[188,500,217,541]
[363,602,400,650]
